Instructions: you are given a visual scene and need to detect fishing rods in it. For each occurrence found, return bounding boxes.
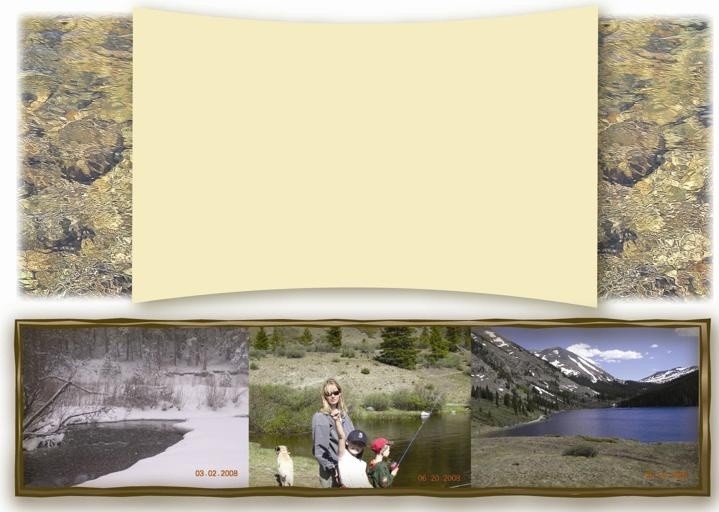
[391,392,444,470]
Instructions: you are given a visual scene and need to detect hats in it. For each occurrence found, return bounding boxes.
[371,438,394,450]
[347,430,367,447]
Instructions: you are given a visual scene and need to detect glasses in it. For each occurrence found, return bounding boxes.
[324,391,339,396]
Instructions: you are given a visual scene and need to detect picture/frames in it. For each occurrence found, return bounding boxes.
[15,316,712,499]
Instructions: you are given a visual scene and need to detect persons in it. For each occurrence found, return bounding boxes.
[331,409,373,488]
[366,438,399,488]
[312,379,354,488]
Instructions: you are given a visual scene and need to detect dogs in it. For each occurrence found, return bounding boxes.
[275,445,293,487]
